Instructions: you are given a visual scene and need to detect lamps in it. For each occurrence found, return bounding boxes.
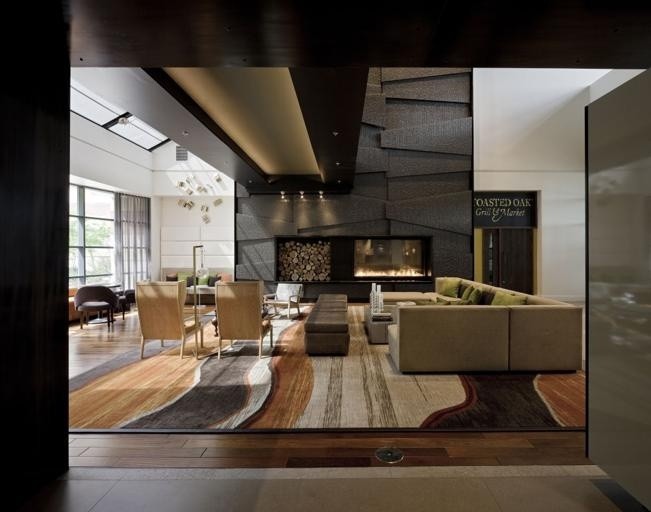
[193,243,209,359]
[177,146,188,162]
[281,191,324,199]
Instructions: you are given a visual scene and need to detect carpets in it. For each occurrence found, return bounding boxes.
[68,305,588,433]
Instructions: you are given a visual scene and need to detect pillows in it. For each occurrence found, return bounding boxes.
[416,299,451,305]
[167,273,233,287]
[463,284,475,299]
[491,291,528,305]
[440,277,462,298]
[470,284,483,303]
[436,297,473,305]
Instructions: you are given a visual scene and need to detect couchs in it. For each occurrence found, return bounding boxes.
[163,267,234,305]
[380,275,584,373]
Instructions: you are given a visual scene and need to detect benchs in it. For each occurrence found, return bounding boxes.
[69,287,110,322]
[304,294,351,356]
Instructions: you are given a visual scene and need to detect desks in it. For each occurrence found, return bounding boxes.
[365,303,397,343]
[88,283,121,324]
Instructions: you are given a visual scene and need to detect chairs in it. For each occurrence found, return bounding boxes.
[117,290,135,320]
[264,283,304,318]
[213,280,273,358]
[136,279,203,359]
[75,285,118,327]
